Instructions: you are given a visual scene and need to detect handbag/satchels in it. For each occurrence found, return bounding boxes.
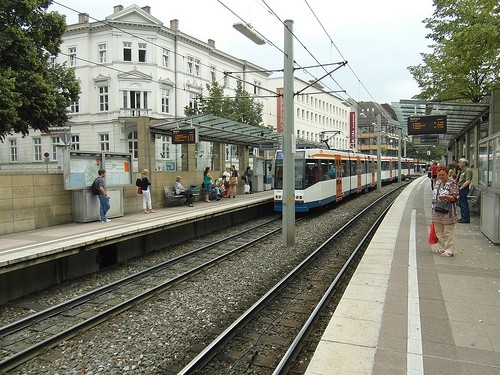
[429,223,438,244]
[434,182,451,214]
[224,180,230,186]
[241,171,246,180]
[201,182,205,189]
[229,177,237,184]
[137,188,142,194]
[243,183,250,194]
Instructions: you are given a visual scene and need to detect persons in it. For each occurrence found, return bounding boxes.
[210,165,237,201]
[174,176,194,207]
[428,165,462,190]
[204,167,212,202]
[94,169,112,223]
[245,167,253,194]
[457,158,472,223]
[306,165,328,184]
[430,167,460,257]
[141,169,156,214]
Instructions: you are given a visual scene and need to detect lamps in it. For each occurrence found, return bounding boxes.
[425,104,433,115]
[372,122,378,127]
[341,101,352,107]
[233,22,268,45]
[360,112,368,118]
[310,79,323,87]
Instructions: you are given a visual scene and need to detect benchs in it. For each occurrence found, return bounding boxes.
[467,185,479,202]
[163,187,199,207]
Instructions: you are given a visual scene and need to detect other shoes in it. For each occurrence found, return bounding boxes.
[100,219,111,223]
[457,219,470,223]
[439,251,454,257]
[143,211,156,214]
[184,201,193,207]
[216,194,223,198]
[246,191,253,194]
[434,248,445,254]
[227,195,230,198]
[216,199,221,201]
[232,196,235,198]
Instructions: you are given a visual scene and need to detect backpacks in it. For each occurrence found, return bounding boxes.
[89,177,102,195]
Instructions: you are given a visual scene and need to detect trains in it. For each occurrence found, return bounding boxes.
[268,130,434,217]
